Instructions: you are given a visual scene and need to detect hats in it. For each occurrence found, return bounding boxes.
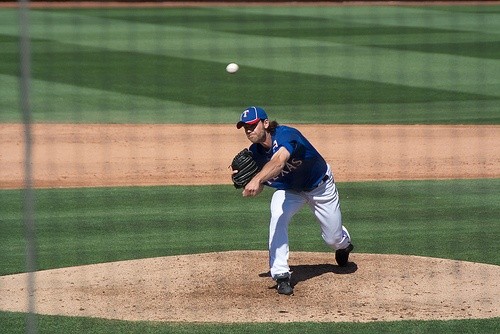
[237,107,268,130]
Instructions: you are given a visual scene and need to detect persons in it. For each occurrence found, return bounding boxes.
[235,105,354,295]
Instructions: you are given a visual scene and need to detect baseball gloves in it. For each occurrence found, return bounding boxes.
[231,148,261,190]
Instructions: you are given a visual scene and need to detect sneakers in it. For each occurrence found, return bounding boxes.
[277,278,293,294]
[334,245,353,266]
